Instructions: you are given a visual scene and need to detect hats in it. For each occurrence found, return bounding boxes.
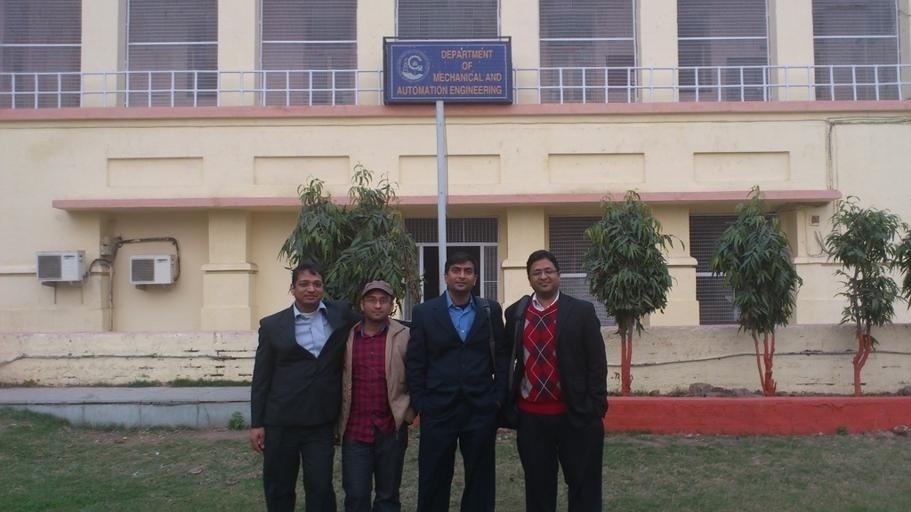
[360,280,396,299]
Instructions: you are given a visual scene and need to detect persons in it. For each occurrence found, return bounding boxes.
[405,251,506,511]
[250,263,360,512]
[504,250,608,511]
[336,280,419,511]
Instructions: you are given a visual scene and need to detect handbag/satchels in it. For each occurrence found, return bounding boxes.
[496,381,520,430]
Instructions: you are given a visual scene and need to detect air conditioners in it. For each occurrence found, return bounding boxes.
[35,250,86,283]
[129,254,174,286]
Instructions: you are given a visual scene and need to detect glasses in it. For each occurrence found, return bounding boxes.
[364,297,392,305]
[528,268,558,278]
